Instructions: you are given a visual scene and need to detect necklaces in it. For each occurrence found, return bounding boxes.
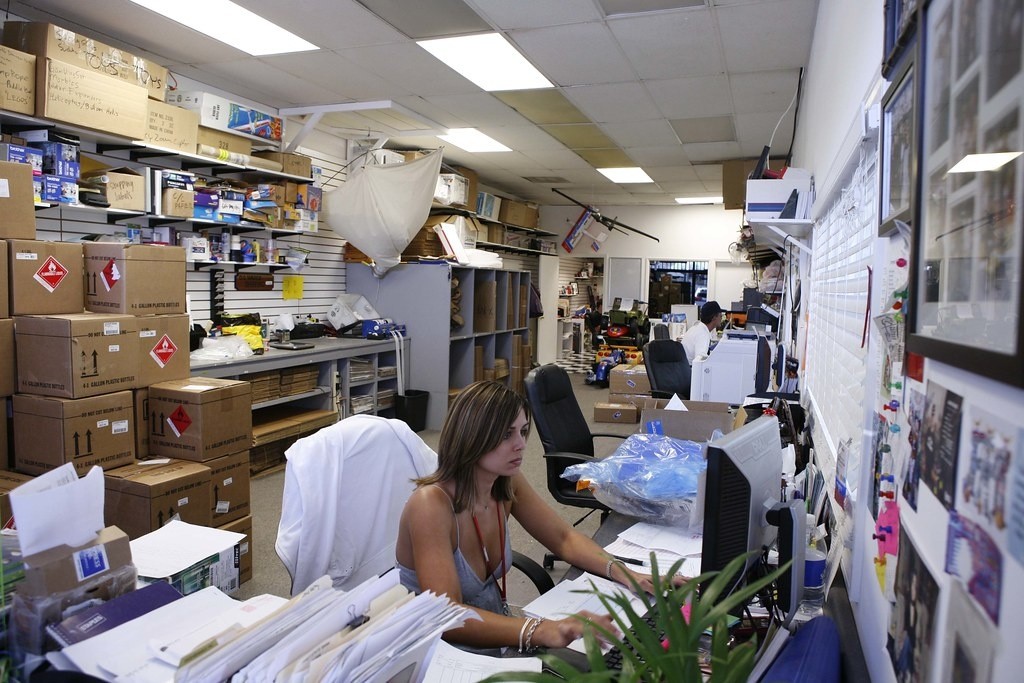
[478,497,493,510]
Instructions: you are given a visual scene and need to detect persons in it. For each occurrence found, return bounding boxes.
[591,299,603,333]
[680,301,722,365]
[395,381,699,655]
[721,311,739,330]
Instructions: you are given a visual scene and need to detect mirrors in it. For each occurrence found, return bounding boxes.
[645,258,711,324]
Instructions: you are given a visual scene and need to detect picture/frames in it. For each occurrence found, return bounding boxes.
[905,0,1024,392]
[877,42,917,239]
[880,0,917,80]
[570,282,579,294]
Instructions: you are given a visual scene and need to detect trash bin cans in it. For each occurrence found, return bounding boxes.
[393,389,429,432]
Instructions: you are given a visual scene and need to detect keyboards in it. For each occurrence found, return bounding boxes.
[603,594,701,673]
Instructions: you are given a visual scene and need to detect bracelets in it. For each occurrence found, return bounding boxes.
[606,558,626,579]
[525,617,547,652]
[517,617,532,653]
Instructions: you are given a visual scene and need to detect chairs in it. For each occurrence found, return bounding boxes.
[522,362,631,571]
[272,413,556,595]
[642,338,691,400]
[654,323,670,339]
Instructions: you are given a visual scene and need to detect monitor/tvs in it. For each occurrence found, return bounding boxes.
[744,319,768,333]
[700,415,807,643]
[755,335,771,395]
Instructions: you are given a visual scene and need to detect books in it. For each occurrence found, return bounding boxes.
[603,523,702,581]
[521,571,663,656]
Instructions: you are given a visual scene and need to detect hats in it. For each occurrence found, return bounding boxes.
[700,300,727,321]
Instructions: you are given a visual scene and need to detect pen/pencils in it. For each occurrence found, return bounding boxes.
[614,555,647,568]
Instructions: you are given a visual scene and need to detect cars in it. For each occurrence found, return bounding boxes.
[694,287,707,309]
[667,272,686,282]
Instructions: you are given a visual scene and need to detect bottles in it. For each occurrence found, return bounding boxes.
[796,514,828,616]
[221,227,260,263]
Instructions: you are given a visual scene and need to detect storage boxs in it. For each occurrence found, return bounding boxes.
[0,20,312,656]
[609,364,652,396]
[671,303,698,330]
[326,293,381,336]
[593,401,637,424]
[350,145,558,381]
[640,398,734,442]
[722,158,789,211]
[746,178,794,224]
[649,273,691,313]
[608,393,652,421]
[669,322,686,341]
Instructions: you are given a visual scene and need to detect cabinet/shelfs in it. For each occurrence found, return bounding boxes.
[475,214,559,258]
[345,262,531,431]
[0,109,316,273]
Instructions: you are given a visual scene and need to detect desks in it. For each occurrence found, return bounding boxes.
[190,336,411,425]
[557,318,585,368]
[559,509,873,683]
